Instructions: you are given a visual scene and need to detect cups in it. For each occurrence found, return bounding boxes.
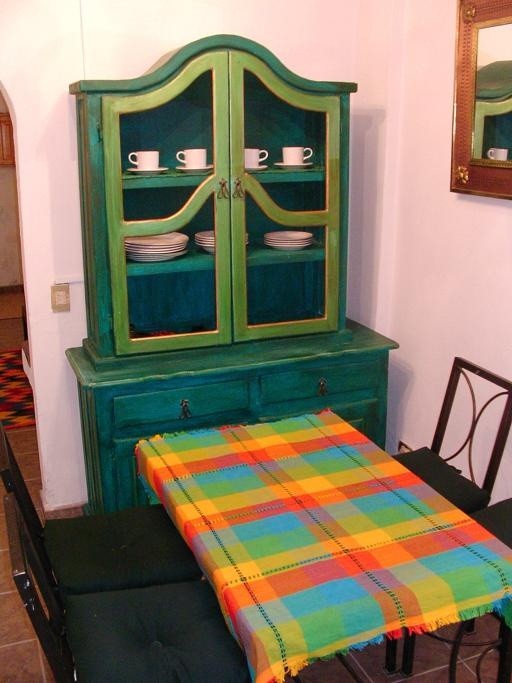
[487,147,508,161]
[175,148,207,168]
[281,146,313,163]
[128,150,160,169]
[245,148,269,167]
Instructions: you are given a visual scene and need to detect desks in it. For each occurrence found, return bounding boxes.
[131,410,511,682]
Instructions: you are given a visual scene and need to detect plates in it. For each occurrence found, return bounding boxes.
[126,167,170,174]
[194,230,249,255]
[244,164,268,171]
[175,164,213,173]
[125,231,190,263]
[273,161,314,169]
[263,230,315,251]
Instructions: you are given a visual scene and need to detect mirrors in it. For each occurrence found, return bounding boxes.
[450,0,512,203]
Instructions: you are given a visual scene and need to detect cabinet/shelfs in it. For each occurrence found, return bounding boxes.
[69,35,341,350]
[84,349,389,507]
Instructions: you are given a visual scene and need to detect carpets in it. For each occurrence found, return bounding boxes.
[2,348,36,433]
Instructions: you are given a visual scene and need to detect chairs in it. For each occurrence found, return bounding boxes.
[1,434,207,596]
[404,494,511,683]
[390,356,511,636]
[3,493,251,682]
